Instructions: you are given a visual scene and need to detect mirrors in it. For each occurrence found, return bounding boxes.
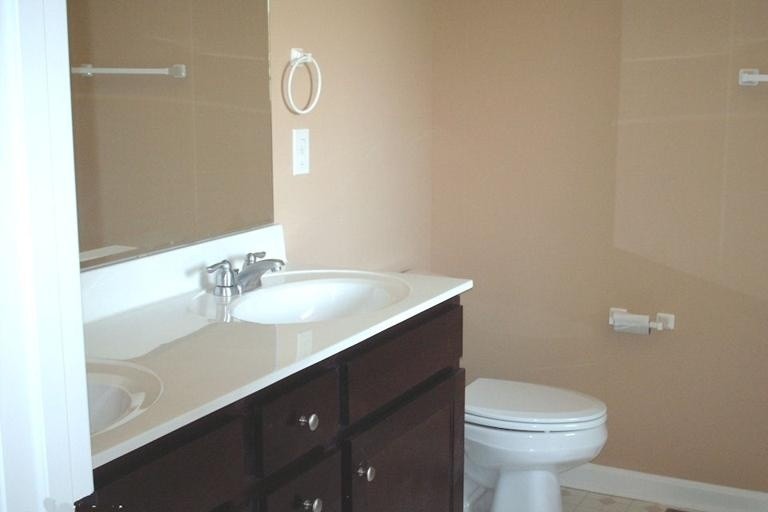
[64,0,276,272]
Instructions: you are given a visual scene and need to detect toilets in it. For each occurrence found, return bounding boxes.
[464,375,608,512]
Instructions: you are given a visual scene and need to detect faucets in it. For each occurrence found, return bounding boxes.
[238,259,284,291]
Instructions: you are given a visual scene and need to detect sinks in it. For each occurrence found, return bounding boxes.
[86,358,166,435]
[228,270,410,324]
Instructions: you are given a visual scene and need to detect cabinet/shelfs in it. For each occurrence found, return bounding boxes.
[346,299,467,511]
